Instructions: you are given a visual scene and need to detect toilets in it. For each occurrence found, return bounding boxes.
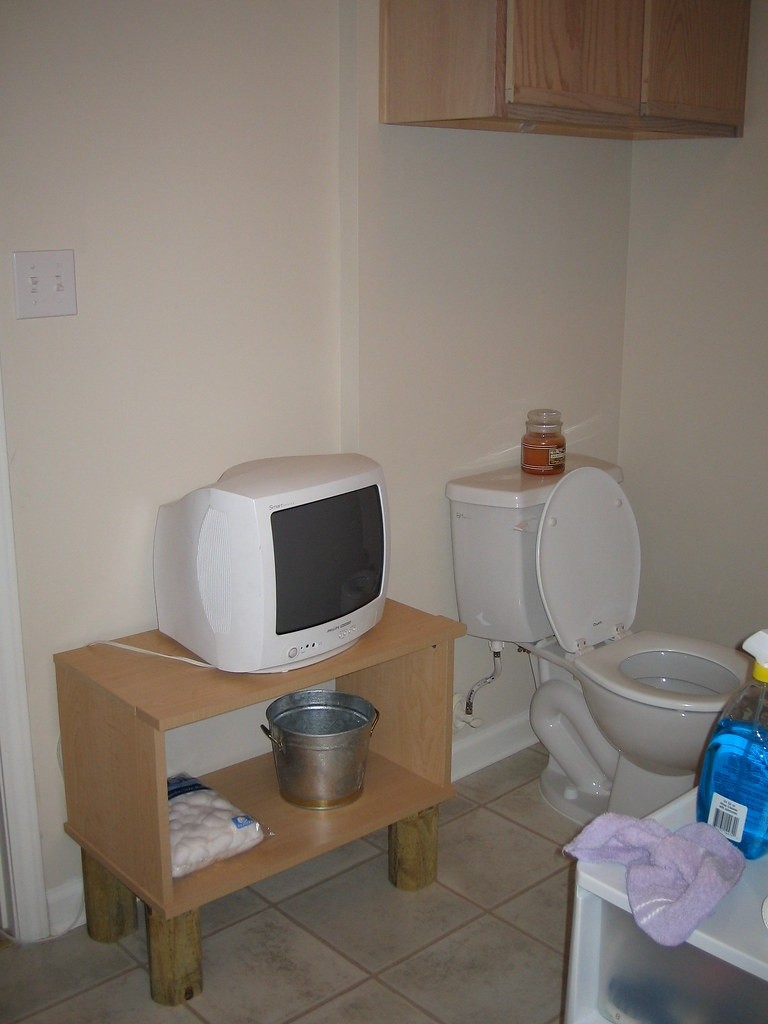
[444,452,755,830]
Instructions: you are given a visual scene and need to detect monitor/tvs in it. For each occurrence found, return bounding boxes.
[152,454,391,673]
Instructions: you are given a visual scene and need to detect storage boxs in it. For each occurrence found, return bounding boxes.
[595,894,768,1024]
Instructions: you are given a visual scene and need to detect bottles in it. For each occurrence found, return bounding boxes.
[521,408,568,476]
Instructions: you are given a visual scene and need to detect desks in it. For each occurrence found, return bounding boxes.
[52,598,467,1008]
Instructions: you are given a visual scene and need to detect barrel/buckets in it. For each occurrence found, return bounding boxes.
[261,690,381,811]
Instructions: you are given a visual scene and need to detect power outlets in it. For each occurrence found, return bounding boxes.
[13,249,77,319]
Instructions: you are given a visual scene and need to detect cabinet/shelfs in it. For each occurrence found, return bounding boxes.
[380,0,755,139]
[553,793,768,1024]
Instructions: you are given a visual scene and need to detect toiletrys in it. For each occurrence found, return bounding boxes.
[694,629,768,862]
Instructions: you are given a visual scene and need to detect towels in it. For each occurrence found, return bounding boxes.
[561,812,746,948]
